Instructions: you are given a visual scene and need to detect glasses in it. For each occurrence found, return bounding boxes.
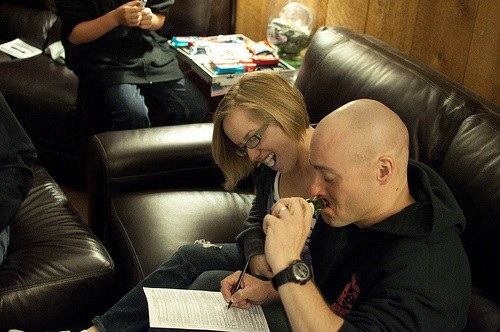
[235,118,270,158]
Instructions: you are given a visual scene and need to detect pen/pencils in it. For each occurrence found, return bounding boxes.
[139,0,147,8]
[227,256,251,310]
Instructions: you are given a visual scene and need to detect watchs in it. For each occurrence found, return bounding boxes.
[271,259,315,291]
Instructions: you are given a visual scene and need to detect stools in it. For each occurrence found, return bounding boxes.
[0,166,116,332]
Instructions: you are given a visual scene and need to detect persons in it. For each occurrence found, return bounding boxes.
[47,0,192,129]
[80,70,354,332]
[220,99,472,332]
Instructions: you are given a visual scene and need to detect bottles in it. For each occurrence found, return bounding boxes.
[305,199,329,215]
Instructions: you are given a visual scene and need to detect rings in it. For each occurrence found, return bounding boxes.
[276,207,288,219]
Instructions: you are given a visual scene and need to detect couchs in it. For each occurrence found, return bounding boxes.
[0,0,236,132]
[87,26,500,332]
